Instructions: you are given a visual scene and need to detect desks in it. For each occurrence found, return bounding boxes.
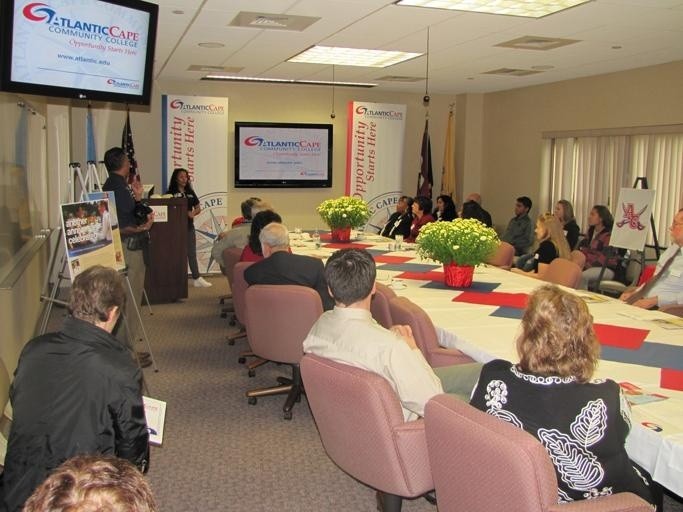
[287,228,683,505]
[143,193,191,303]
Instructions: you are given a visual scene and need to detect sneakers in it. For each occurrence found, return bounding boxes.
[194,276,212,288]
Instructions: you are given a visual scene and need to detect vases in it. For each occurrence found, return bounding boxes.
[331,226,350,241]
[442,264,473,288]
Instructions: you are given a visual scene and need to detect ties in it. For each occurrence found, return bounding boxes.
[622,248,681,303]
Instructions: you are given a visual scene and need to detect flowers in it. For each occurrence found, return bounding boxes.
[315,196,373,230]
[414,217,502,269]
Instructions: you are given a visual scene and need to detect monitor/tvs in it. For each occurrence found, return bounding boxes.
[234,121,333,189]
[0,0,159,105]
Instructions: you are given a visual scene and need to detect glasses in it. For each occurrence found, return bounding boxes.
[671,220,683,228]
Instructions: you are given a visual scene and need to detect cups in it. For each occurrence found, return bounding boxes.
[388,243,395,252]
[294,223,303,235]
[395,235,403,251]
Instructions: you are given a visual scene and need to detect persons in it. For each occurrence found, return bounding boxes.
[242,223,336,313]
[102,148,154,367]
[576,204,619,292]
[433,195,458,222]
[300,249,447,420]
[214,198,294,268]
[452,192,495,231]
[164,167,212,287]
[468,285,664,511]
[501,197,535,256]
[514,211,570,272]
[553,200,580,252]
[0,266,149,511]
[378,196,414,239]
[405,197,435,243]
[22,456,156,511]
[620,208,683,311]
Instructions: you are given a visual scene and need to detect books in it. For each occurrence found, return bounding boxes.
[142,395,169,449]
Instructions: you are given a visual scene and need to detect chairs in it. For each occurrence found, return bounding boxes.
[298,354,436,512]
[594,247,646,299]
[369,281,397,328]
[229,261,254,363]
[482,239,515,269]
[244,285,325,421]
[389,296,474,369]
[221,247,244,328]
[538,259,582,289]
[568,248,586,267]
[423,395,658,512]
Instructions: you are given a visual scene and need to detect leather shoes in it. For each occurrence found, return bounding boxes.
[134,349,153,367]
